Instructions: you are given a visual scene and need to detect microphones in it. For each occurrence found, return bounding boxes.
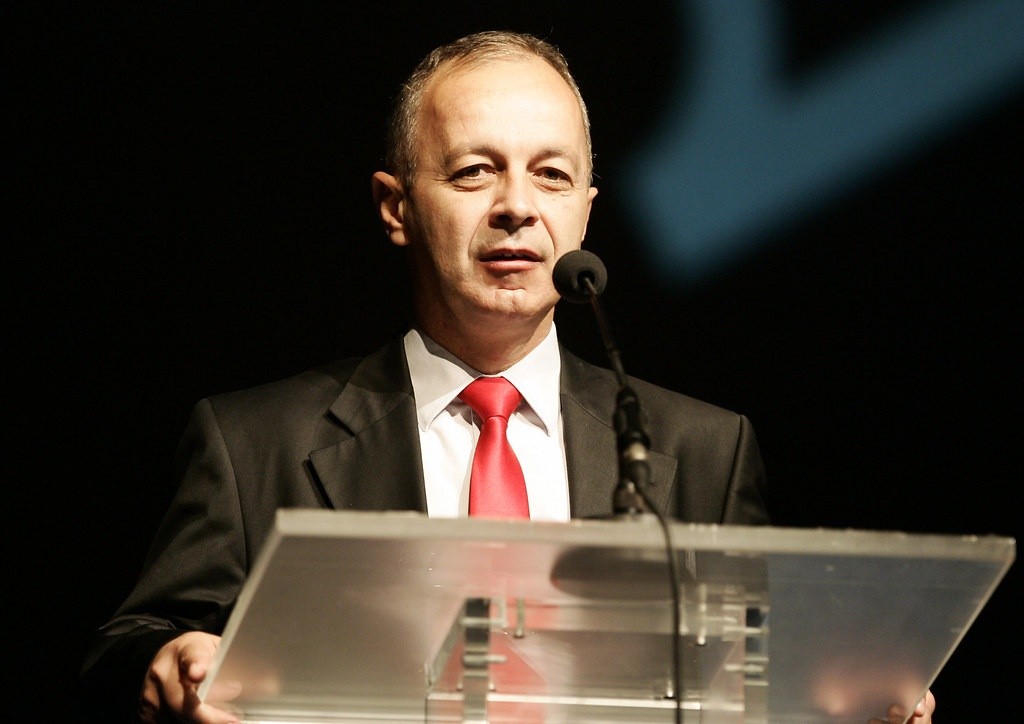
[551,250,653,492]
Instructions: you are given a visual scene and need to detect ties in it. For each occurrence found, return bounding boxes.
[433,377,557,718]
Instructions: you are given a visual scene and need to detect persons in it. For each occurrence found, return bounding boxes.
[79,32,936,723]
